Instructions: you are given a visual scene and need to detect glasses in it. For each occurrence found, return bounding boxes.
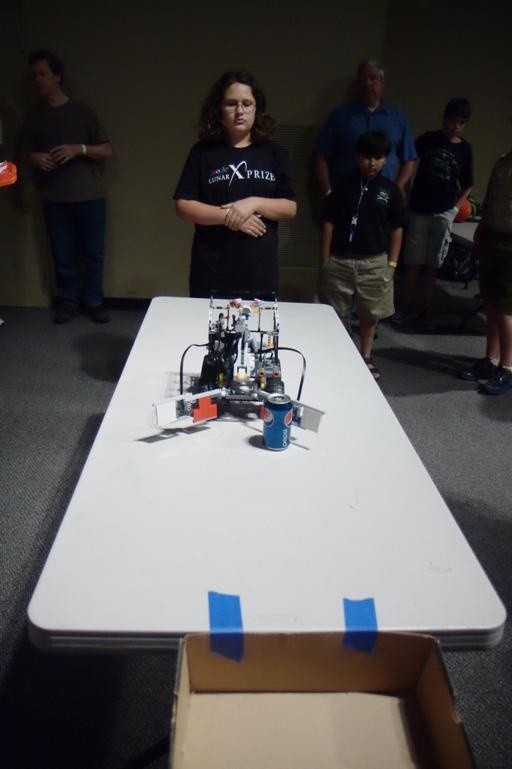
[221,99,255,115]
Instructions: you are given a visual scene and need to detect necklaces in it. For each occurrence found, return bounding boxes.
[349,177,368,242]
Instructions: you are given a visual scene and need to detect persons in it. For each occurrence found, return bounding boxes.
[320,132,405,382]
[173,69,298,302]
[391,96,474,332]
[313,61,418,201]
[461,148,512,395]
[16,49,113,324]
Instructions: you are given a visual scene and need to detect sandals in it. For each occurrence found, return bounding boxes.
[364,357,380,381]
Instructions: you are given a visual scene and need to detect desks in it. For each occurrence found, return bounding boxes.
[447,223,488,333]
[24,294,507,651]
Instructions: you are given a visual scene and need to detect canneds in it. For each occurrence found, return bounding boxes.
[263,392,293,451]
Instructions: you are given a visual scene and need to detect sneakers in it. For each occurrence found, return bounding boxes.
[392,311,416,323]
[483,363,512,395]
[459,358,498,381]
[53,306,74,324]
[76,304,110,323]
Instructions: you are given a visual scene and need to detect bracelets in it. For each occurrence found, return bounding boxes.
[323,189,332,197]
[80,143,87,156]
[389,261,397,268]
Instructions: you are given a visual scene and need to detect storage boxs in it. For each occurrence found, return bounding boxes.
[168,634,477,769]
